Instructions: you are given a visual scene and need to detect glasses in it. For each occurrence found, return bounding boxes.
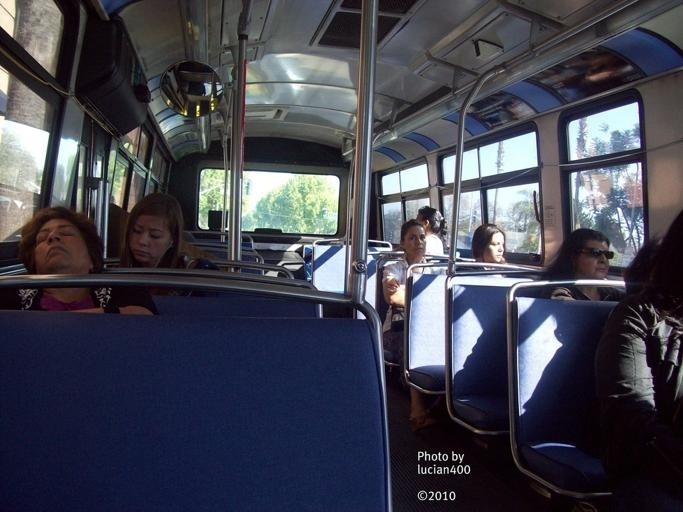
[580,249,614,259]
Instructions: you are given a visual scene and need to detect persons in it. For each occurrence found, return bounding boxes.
[381,206,447,429]
[118,192,220,297]
[544,229,625,302]
[598,212,680,511]
[456,223,520,277]
[0,206,159,315]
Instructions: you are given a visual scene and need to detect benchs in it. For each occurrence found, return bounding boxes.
[0,229,654,511]
